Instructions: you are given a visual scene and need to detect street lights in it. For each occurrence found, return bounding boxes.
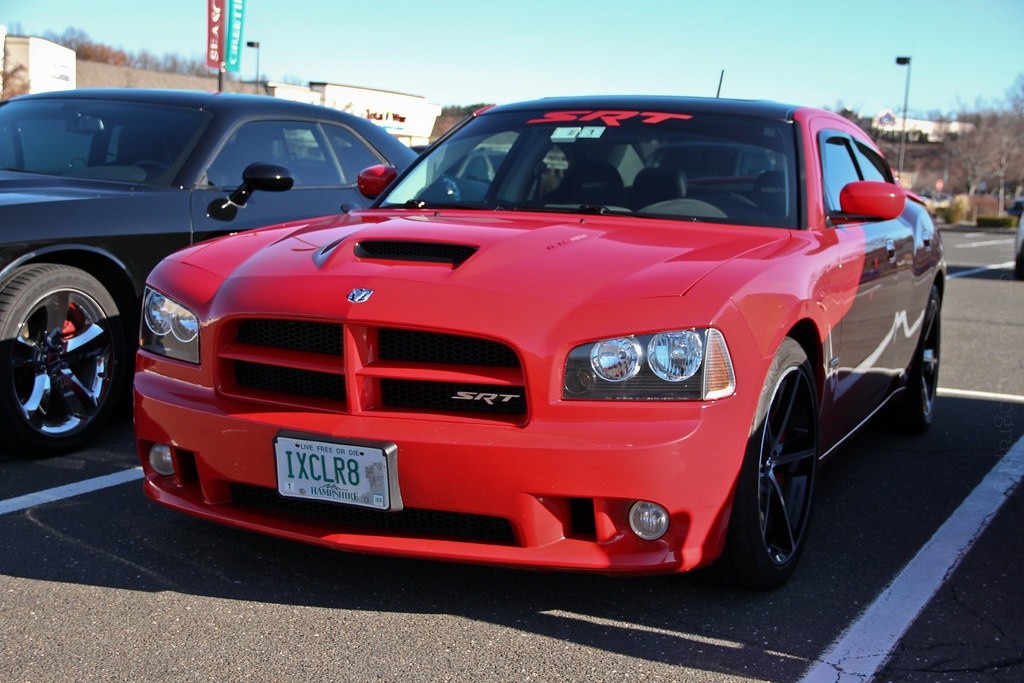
[248,42,260,95]
[897,57,911,180]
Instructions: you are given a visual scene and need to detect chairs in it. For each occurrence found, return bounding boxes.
[118,127,171,184]
[533,161,626,208]
[752,171,789,223]
[631,167,683,208]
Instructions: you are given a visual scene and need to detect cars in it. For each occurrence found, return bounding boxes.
[0,89,493,461]
[133,95,946,594]
